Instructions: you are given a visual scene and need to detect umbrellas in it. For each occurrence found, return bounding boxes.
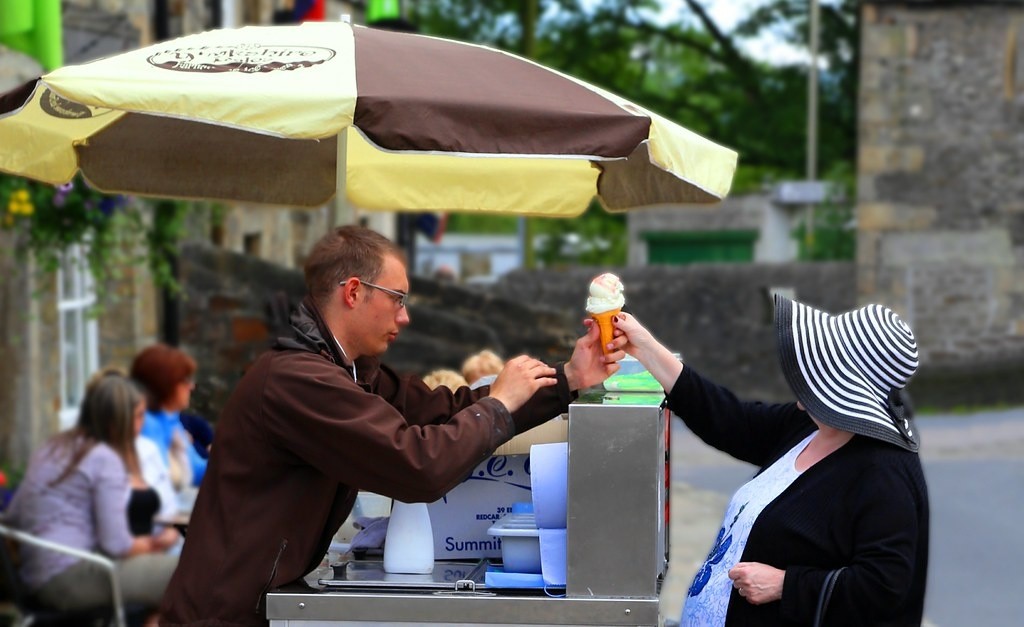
[3,21,742,526]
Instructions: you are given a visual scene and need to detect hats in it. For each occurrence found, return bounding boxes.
[768,290,927,452]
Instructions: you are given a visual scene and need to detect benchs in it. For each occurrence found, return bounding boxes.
[0,516,166,627]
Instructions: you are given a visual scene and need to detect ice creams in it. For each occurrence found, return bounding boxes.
[585,273,626,364]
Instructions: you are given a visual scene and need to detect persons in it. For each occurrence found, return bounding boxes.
[1,341,218,626]
[581,279,930,627]
[151,225,631,627]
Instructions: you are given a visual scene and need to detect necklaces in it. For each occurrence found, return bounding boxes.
[332,333,359,387]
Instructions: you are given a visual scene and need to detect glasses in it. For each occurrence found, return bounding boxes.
[175,375,197,386]
[337,279,409,308]
